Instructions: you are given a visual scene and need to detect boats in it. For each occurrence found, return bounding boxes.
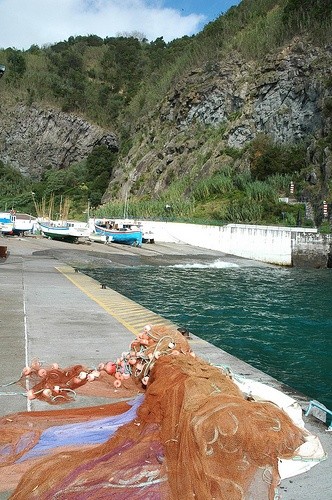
[95,220,142,247]
[0,212,32,237]
[33,214,92,244]
